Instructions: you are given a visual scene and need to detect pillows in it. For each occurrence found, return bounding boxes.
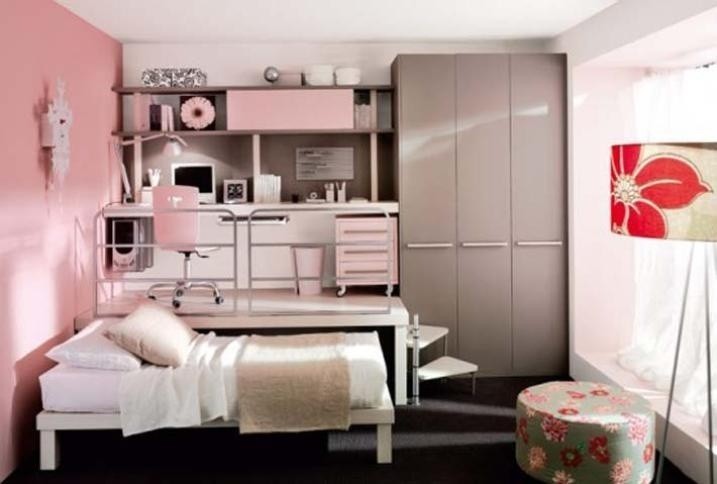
[43,305,205,371]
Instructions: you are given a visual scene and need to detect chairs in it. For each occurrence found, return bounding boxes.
[144,185,226,311]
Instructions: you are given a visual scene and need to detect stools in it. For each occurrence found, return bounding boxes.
[515,381,655,483]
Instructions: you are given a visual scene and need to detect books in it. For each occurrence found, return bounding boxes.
[261,174,281,203]
[149,104,175,131]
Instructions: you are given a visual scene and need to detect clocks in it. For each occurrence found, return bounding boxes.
[42,79,75,191]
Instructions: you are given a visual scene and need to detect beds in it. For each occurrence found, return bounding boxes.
[36,329,400,470]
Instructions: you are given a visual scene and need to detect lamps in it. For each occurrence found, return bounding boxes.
[611,140,716,484]
[117,132,188,159]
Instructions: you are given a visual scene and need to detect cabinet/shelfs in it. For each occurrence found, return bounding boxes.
[110,83,397,137]
[391,49,568,382]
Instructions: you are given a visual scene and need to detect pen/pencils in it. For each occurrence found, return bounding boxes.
[324,182,348,190]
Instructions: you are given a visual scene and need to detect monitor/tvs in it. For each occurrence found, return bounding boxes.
[171,162,217,204]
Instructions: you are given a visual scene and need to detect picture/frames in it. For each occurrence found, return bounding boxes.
[223,177,248,204]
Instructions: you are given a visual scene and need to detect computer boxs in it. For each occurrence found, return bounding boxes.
[112,219,138,272]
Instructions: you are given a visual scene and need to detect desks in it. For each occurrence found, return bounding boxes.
[106,199,397,219]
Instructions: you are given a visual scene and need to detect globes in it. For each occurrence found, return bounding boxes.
[263,66,279,87]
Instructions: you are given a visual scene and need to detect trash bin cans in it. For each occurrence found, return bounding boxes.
[290,244,327,297]
